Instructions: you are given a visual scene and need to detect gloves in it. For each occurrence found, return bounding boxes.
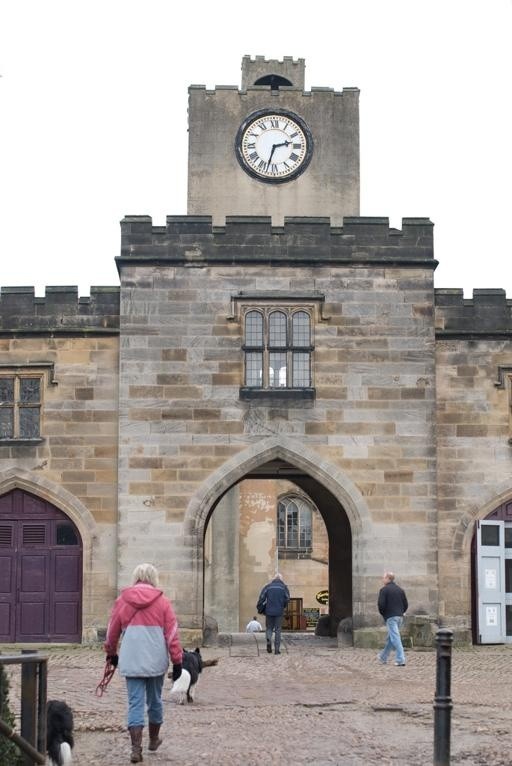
[106,654,118,668]
[173,664,181,681]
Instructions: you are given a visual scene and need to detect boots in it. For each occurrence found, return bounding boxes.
[129,726,142,762]
[148,723,162,750]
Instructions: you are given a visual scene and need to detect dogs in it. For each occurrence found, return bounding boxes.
[169,647,203,706]
[44,699,75,766]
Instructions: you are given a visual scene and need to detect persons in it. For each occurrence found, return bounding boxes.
[104,564,184,764]
[256,573,291,654]
[378,572,408,665]
[246,616,262,633]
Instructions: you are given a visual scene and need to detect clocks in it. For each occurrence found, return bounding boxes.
[232,106,314,183]
